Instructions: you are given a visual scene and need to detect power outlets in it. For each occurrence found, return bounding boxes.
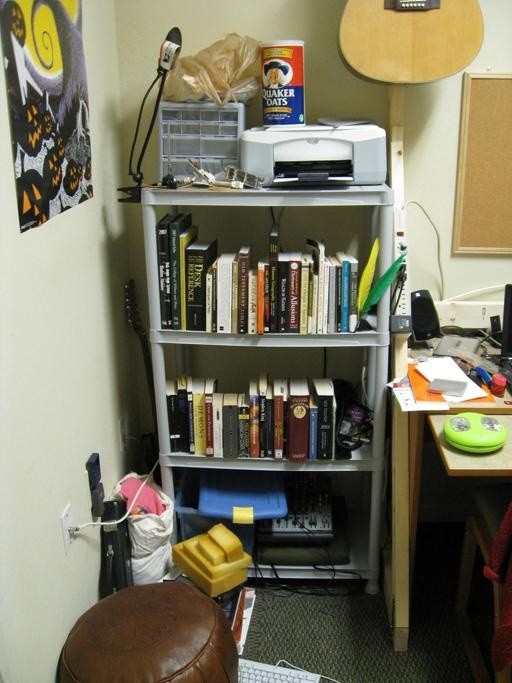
[58,499,81,545]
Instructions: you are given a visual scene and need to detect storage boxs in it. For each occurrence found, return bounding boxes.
[172,472,287,559]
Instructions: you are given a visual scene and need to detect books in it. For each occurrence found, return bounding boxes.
[167,375,335,461]
[158,214,361,334]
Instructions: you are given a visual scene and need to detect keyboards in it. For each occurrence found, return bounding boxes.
[237,657,321,683]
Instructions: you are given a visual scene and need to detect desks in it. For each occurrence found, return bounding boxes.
[380,323,511,653]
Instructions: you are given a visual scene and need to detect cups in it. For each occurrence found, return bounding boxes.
[259,37,306,126]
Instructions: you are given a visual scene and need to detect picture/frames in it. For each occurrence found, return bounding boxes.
[451,69,512,260]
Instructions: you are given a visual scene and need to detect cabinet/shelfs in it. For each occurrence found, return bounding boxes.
[137,178,398,596]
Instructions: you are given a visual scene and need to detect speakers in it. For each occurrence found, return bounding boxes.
[407,289,441,348]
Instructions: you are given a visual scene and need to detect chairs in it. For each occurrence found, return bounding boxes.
[451,482,511,683]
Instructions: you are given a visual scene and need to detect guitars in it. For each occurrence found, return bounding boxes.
[124,277,159,477]
[336,2,485,83]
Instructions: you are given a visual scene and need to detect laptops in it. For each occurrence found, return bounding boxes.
[499,284,511,398]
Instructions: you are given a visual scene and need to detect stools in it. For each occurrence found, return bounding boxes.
[56,577,242,682]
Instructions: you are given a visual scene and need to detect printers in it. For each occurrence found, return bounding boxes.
[239,121,387,187]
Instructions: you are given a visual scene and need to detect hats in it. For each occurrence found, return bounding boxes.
[263,62,289,73]
